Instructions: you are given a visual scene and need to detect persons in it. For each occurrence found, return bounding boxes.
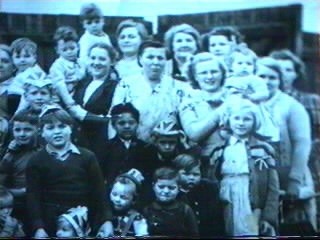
[2,1,319,239]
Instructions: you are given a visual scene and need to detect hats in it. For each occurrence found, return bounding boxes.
[24,71,53,88]
[152,120,180,136]
[116,167,146,191]
[59,204,92,239]
[37,101,64,120]
[79,3,99,16]
